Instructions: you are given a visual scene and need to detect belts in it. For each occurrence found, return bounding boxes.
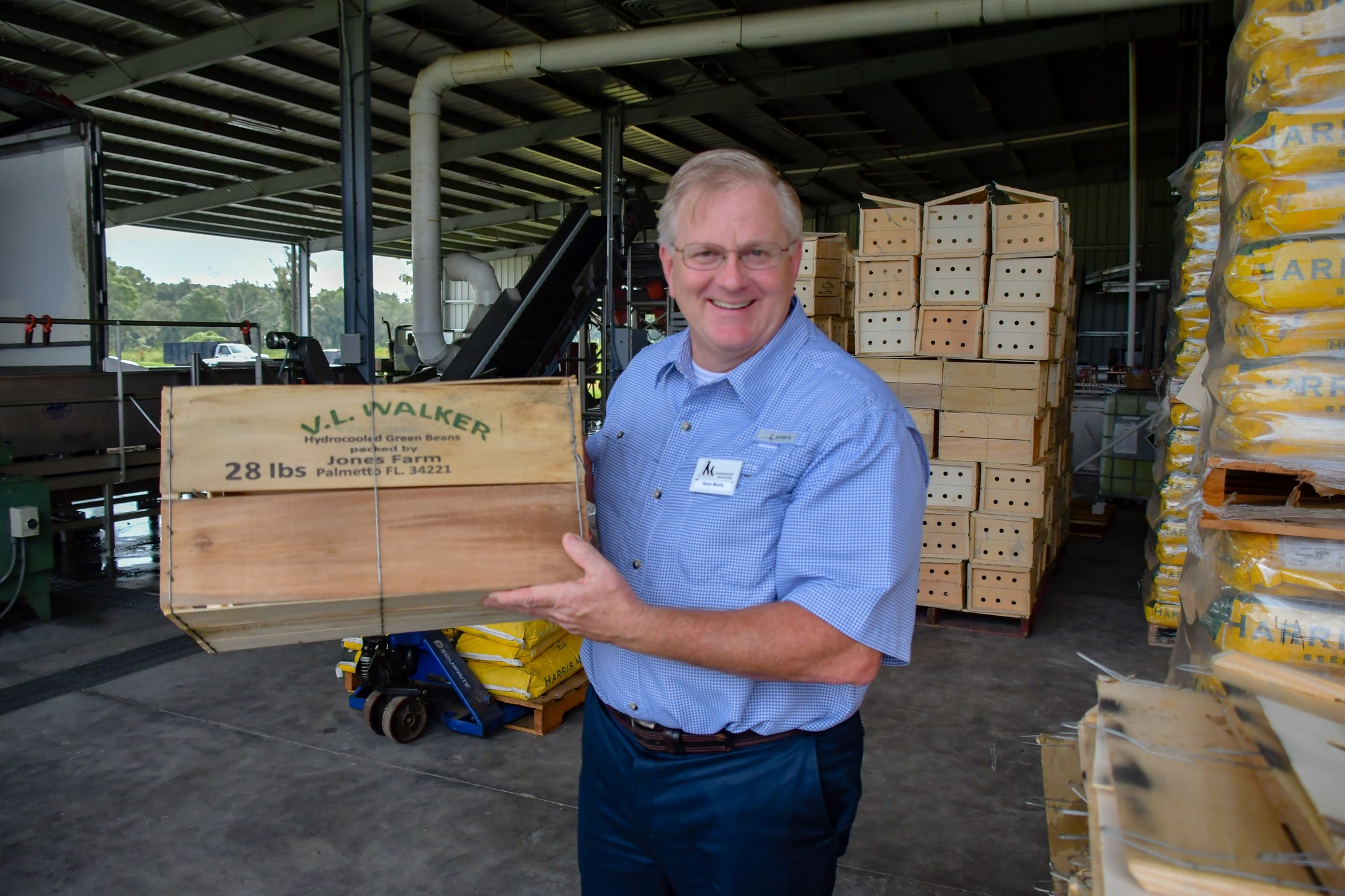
[593,693,802,754]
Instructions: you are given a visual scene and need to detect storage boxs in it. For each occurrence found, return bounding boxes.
[154,375,592,653]
[789,183,1079,618]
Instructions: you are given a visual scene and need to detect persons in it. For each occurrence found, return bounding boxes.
[475,144,929,895]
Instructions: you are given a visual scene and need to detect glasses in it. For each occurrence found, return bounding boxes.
[672,242,796,269]
